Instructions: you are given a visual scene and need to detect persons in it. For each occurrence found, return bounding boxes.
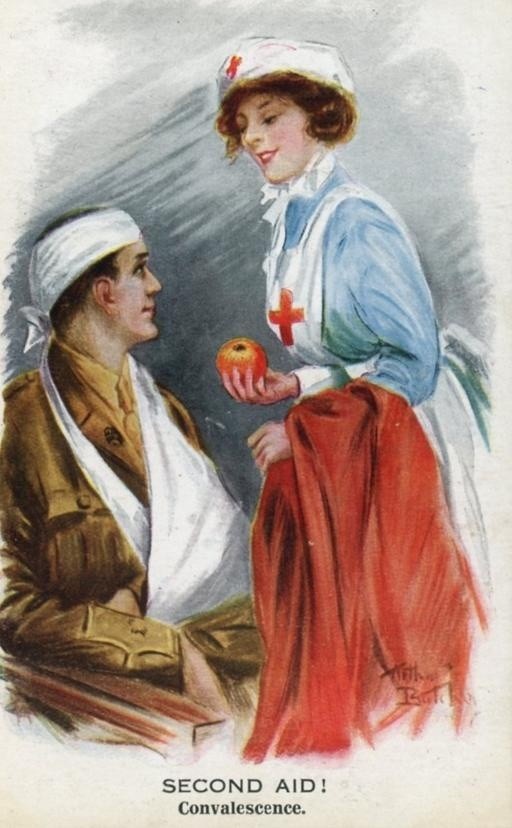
[0,206,266,765]
[213,36,492,765]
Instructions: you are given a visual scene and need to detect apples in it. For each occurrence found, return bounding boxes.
[215,338,267,386]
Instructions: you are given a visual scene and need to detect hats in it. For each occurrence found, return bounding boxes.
[212,33,356,107]
